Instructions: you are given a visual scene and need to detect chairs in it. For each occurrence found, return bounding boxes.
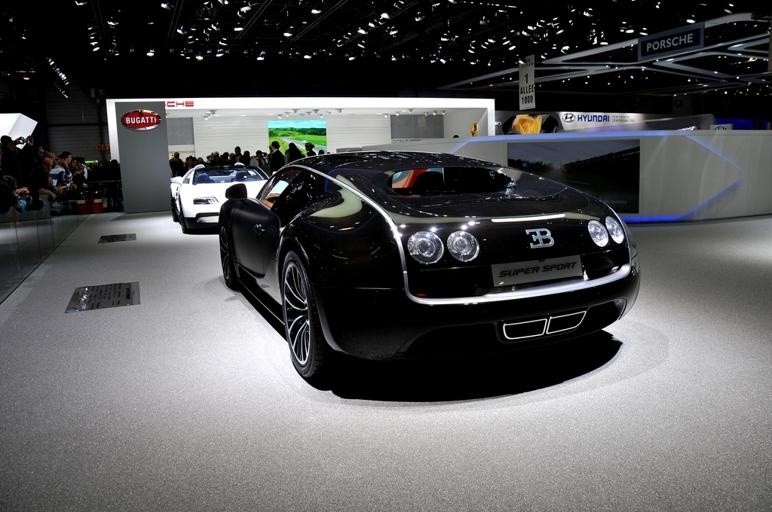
[413,174,447,193]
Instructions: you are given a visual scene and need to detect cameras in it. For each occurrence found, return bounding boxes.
[22,139,28,143]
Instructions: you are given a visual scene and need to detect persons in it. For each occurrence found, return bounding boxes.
[0,133,125,227]
[169,139,325,182]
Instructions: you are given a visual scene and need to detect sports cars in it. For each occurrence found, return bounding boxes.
[218,150,642,381]
[170,161,290,234]
